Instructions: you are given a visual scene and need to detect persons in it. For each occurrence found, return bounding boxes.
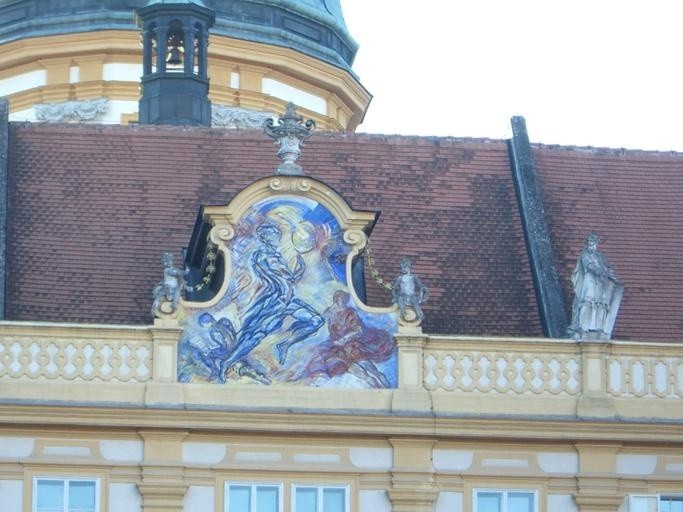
[151,252,193,315]
[567,234,625,338]
[303,290,399,389]
[392,259,429,322]
[198,313,271,384]
[219,225,325,382]
